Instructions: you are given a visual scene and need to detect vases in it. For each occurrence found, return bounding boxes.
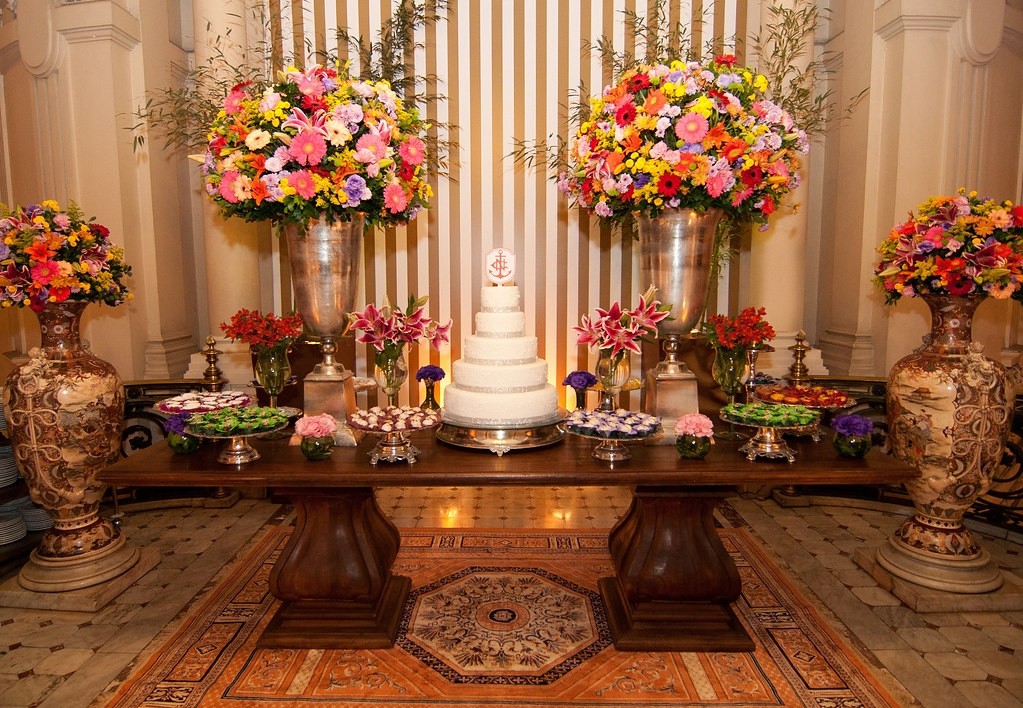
[253,347,294,441]
[2,298,140,568]
[372,344,411,440]
[595,346,632,395]
[710,345,750,440]
[421,379,441,412]
[675,434,709,459]
[301,436,335,462]
[875,292,1015,595]
[282,212,367,447]
[631,209,722,445]
[573,388,586,410]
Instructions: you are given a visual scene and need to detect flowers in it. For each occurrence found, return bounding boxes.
[0,199,134,312]
[562,283,776,445]
[115,1,463,240]
[498,0,871,300]
[219,294,453,439]
[870,189,1023,306]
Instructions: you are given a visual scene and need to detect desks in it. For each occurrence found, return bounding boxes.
[94,431,922,653]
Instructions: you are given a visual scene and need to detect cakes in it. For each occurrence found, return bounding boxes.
[443,248,558,427]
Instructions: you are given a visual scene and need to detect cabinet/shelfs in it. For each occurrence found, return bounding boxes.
[0,429,47,576]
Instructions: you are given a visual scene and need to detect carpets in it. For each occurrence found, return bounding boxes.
[85,526,905,708]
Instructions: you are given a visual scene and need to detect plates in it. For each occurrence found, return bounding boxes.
[0,495,54,546]
[0,402,7,430]
[0,445,24,489]
